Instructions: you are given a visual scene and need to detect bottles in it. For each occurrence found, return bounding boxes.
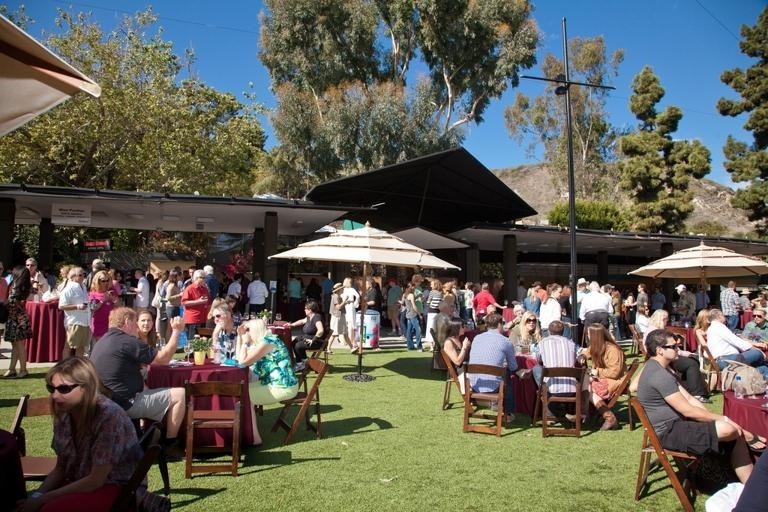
[734,376,745,400]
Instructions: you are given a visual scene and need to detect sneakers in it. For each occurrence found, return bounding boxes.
[696,396,711,402]
[3,369,16,376]
[350,346,358,353]
[326,350,333,355]
[503,412,515,423]
[16,370,28,378]
[491,402,498,411]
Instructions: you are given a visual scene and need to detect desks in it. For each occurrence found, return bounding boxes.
[25,300,65,362]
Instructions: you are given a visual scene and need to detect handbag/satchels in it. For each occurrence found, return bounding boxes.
[152,283,162,308]
[719,359,764,395]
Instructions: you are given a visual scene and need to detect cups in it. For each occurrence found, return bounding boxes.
[225,340,236,361]
[748,368,761,400]
[276,314,281,328]
[183,340,193,367]
[244,312,249,321]
[156,338,165,351]
[250,312,256,320]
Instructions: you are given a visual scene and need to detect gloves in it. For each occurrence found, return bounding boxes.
[77,303,87,310]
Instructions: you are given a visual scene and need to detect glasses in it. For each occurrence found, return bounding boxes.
[662,343,677,350]
[78,271,86,276]
[102,279,108,282]
[526,318,536,323]
[246,328,249,331]
[212,314,221,320]
[752,315,762,318]
[27,264,35,267]
[46,383,79,394]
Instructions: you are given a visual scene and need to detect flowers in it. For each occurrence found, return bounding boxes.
[191,334,212,352]
[257,308,272,319]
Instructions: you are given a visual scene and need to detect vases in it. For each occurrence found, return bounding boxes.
[194,351,206,365]
[262,316,269,324]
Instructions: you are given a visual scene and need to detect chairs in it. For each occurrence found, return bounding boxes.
[184,379,245,478]
[270,354,329,444]
[631,395,700,512]
[307,329,333,359]
[628,324,647,357]
[430,327,474,414]
[462,362,508,436]
[111,420,163,512]
[533,364,586,438]
[596,358,640,431]
[10,393,61,482]
[696,331,726,390]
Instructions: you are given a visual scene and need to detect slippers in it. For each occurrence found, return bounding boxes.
[746,431,768,452]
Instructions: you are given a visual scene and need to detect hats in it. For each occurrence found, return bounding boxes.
[93,260,105,267]
[331,282,345,292]
[675,284,686,290]
[577,278,588,284]
[740,288,752,294]
[604,284,615,290]
[532,281,542,286]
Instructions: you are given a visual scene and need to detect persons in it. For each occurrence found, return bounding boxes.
[135,307,157,350]
[467,313,518,424]
[634,304,650,335]
[411,274,424,326]
[180,269,212,340]
[611,287,623,340]
[650,286,666,316]
[643,309,714,404]
[57,267,92,360]
[339,277,359,348]
[89,307,186,459]
[152,270,168,343]
[471,281,508,324]
[752,293,768,309]
[626,241,764,311]
[0,262,11,359]
[14,355,147,512]
[578,280,614,330]
[558,285,573,340]
[624,289,637,336]
[604,284,618,342]
[425,280,444,353]
[286,274,301,323]
[692,308,711,350]
[540,283,564,338]
[165,272,185,347]
[202,264,220,302]
[463,281,475,324]
[129,270,150,310]
[284,300,330,374]
[532,321,578,424]
[526,280,542,297]
[580,322,628,432]
[304,277,322,311]
[675,284,697,325]
[706,309,768,384]
[226,273,242,303]
[224,294,241,324]
[326,283,360,355]
[719,279,742,331]
[3,267,33,379]
[363,278,377,310]
[182,265,197,291]
[25,258,49,303]
[576,277,590,347]
[383,278,403,337]
[638,328,768,486]
[740,307,768,350]
[89,270,122,350]
[630,283,649,316]
[210,303,241,360]
[320,273,334,314]
[738,286,751,311]
[443,317,472,396]
[507,311,542,345]
[85,258,106,290]
[247,273,269,315]
[396,282,423,351]
[230,317,301,449]
[697,285,710,309]
[107,268,125,297]
[437,282,455,350]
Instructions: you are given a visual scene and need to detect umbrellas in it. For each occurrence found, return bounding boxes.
[267,220,461,380]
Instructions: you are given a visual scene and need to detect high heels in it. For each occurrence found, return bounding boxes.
[566,411,588,423]
[593,419,618,430]
